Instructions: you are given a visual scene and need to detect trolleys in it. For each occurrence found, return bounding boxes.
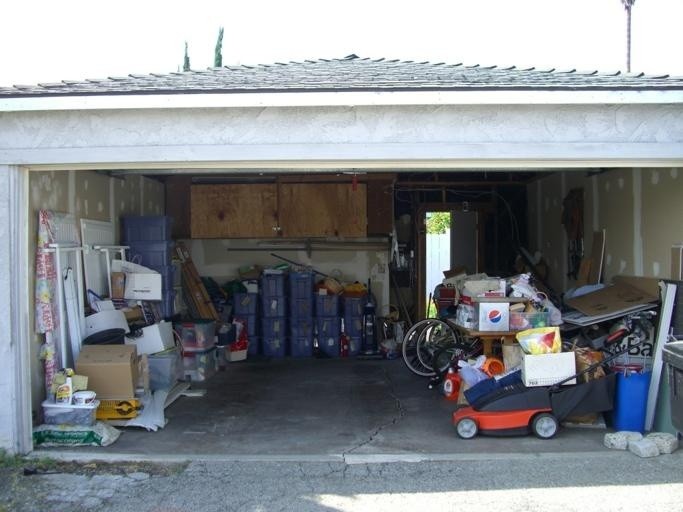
[451,316,653,440]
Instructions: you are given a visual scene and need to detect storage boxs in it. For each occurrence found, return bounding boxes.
[216,346,231,372]
[247,337,260,355]
[42,397,99,426]
[349,340,362,356]
[290,300,312,319]
[146,352,182,390]
[261,276,284,297]
[77,344,138,399]
[318,318,340,338]
[180,349,216,381]
[262,298,286,317]
[123,215,172,241]
[155,292,174,319]
[318,338,340,357]
[261,318,287,338]
[291,340,312,356]
[175,318,215,349]
[344,316,364,338]
[344,297,365,316]
[290,320,313,339]
[520,349,577,387]
[234,292,257,314]
[316,296,337,316]
[263,338,287,357]
[235,314,256,336]
[288,273,311,298]
[155,269,173,290]
[125,243,171,268]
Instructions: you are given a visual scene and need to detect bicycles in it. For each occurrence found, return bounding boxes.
[402,312,508,384]
